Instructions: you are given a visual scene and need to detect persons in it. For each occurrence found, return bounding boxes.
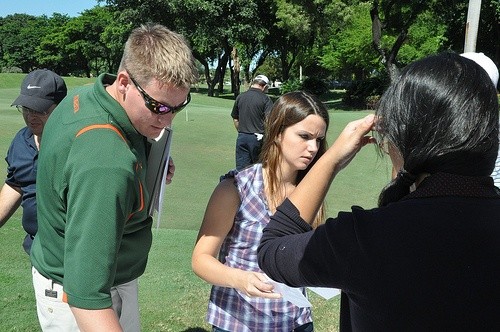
[231,75,274,172]
[192,90,329,331]
[30,22,192,332]
[1,68,68,255]
[257,53,500,332]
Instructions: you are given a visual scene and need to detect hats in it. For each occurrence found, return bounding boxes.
[254,75,269,93]
[458,52,500,91]
[11,69,67,113]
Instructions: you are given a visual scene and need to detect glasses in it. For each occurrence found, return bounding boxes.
[127,70,191,115]
[16,105,57,116]
